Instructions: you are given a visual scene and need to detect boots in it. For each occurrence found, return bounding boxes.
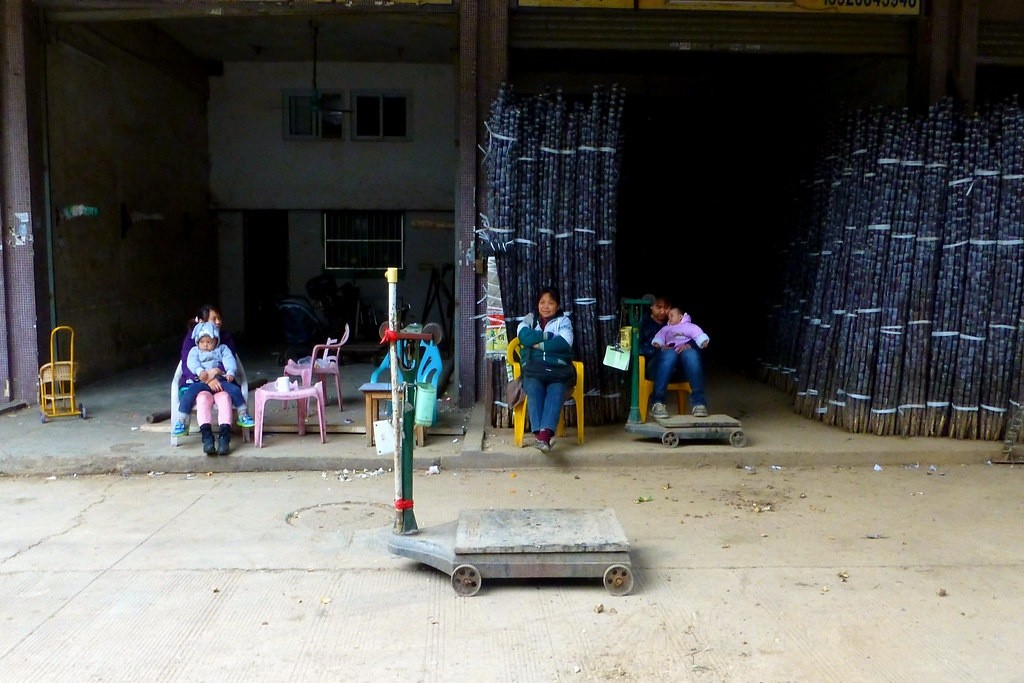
[199,423,215,453]
[217,424,231,454]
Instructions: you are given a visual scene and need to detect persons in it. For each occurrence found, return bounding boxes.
[516,287,573,454]
[639,292,709,418]
[172,303,256,455]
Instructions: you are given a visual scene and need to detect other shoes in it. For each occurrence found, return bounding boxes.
[549,438,557,449]
[534,438,551,455]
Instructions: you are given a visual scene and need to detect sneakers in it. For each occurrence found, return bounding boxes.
[691,404,708,416]
[236,413,255,427]
[648,402,669,418]
[172,415,190,437]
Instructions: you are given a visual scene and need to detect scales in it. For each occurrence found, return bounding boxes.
[384,265,636,599]
[618,298,748,447]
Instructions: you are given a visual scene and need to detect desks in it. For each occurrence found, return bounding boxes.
[254,381,327,448]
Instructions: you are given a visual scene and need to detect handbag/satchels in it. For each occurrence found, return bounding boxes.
[505,377,525,410]
[524,359,577,386]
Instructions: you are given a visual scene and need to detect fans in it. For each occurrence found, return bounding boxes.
[261,22,351,113]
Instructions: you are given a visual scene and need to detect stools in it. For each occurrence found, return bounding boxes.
[358,382,425,448]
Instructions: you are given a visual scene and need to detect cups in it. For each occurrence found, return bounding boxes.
[275,376,290,393]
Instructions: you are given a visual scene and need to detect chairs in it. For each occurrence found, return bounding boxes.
[639,356,692,423]
[281,323,350,410]
[370,340,443,425]
[170,352,251,444]
[506,337,584,448]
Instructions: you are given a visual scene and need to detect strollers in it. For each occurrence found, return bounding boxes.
[37,325,88,423]
